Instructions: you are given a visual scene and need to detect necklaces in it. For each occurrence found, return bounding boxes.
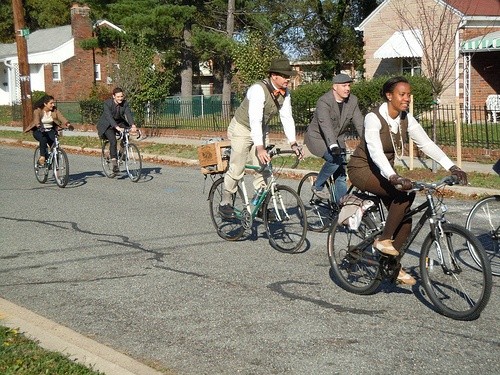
[384,108,403,161]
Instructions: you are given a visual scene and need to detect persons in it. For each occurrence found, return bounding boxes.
[24,96,74,172]
[346,76,468,285]
[305,74,365,211]
[218,58,305,224]
[98,88,137,173]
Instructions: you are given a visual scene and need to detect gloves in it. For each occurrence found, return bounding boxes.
[448,164,468,185]
[332,147,346,156]
[38,125,46,132]
[390,175,416,191]
[69,125,74,131]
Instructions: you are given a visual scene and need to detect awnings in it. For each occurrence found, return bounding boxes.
[374,31,424,59]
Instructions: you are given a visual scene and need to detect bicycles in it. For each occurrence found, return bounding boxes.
[33,127,71,189]
[100,129,143,183]
[326,174,493,322]
[296,149,388,239]
[202,144,307,255]
[463,195,500,279]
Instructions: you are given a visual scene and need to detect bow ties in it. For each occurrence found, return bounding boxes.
[273,88,286,96]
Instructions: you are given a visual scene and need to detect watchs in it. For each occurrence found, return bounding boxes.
[292,142,299,149]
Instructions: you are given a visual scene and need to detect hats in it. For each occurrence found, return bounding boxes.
[264,58,297,76]
[332,74,353,84]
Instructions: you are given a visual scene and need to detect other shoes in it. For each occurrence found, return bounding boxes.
[119,151,128,161]
[311,185,329,199]
[218,203,237,218]
[257,208,278,221]
[39,156,46,165]
[113,165,119,172]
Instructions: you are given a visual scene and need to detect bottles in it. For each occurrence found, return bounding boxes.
[368,207,383,229]
[250,186,264,206]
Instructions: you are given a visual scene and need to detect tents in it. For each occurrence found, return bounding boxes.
[460,31,500,50]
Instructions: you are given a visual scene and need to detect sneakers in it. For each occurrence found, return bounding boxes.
[373,238,400,256]
[397,266,417,285]
